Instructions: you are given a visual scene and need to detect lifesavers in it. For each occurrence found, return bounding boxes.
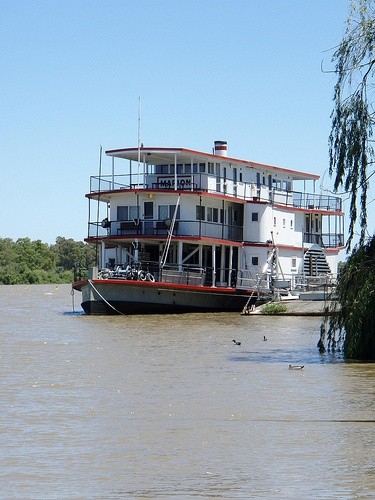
[146,272,155,283]
[137,269,147,281]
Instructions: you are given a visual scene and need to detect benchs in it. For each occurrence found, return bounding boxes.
[117,222,143,236]
[152,221,179,236]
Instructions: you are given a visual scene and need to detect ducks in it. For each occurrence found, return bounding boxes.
[232,339,241,345]
[262,335,268,341]
[289,364,304,370]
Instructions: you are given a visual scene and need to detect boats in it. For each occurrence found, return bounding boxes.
[69,138,344,315]
